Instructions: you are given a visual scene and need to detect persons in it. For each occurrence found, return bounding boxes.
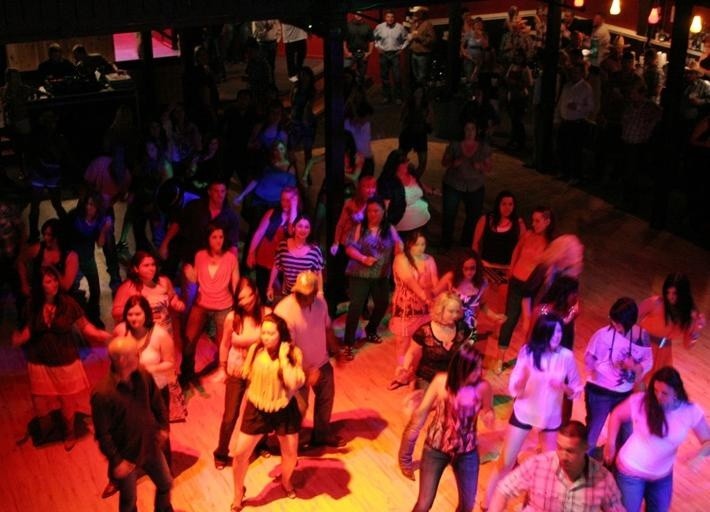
[450,87,500,141]
[285,67,326,185]
[558,8,580,65]
[662,66,710,178]
[537,235,583,351]
[182,223,240,378]
[213,275,271,471]
[386,231,439,365]
[501,5,547,61]
[388,290,471,426]
[339,95,376,177]
[343,130,366,186]
[247,186,299,304]
[398,84,436,178]
[330,175,377,321]
[685,29,710,68]
[397,346,494,512]
[79,149,134,293]
[34,44,73,94]
[636,272,706,391]
[101,295,176,499]
[145,178,182,274]
[407,6,436,104]
[461,14,473,59]
[610,36,668,79]
[254,18,283,97]
[90,335,175,512]
[185,136,226,197]
[0,200,14,255]
[432,251,508,346]
[234,135,299,206]
[547,65,594,178]
[582,11,610,75]
[4,68,55,150]
[134,136,173,253]
[585,296,653,467]
[68,191,114,329]
[531,51,567,170]
[72,44,119,88]
[266,214,326,308]
[503,47,532,139]
[158,179,240,281]
[342,195,403,360]
[603,366,710,512]
[377,148,443,253]
[608,82,661,185]
[373,9,411,105]
[111,251,188,422]
[10,266,113,450]
[463,18,489,83]
[189,44,220,137]
[480,312,583,512]
[27,114,67,246]
[283,22,307,84]
[440,121,493,251]
[272,269,346,446]
[472,191,527,334]
[17,218,80,295]
[146,106,178,167]
[488,420,628,512]
[244,102,288,191]
[494,203,554,376]
[342,11,375,95]
[230,313,306,512]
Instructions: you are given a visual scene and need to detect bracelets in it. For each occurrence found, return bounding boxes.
[432,186,436,195]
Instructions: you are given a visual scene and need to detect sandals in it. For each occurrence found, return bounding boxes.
[344,345,353,360]
[367,333,382,343]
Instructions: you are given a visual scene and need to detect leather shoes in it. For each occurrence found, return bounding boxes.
[102,483,118,500]
[214,456,226,470]
[322,434,346,446]
[256,445,270,458]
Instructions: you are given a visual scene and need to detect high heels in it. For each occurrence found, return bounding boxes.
[281,481,295,499]
[230,486,246,512]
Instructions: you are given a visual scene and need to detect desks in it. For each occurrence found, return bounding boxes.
[6,74,128,112]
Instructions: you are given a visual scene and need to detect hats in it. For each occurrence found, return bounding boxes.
[291,271,318,296]
